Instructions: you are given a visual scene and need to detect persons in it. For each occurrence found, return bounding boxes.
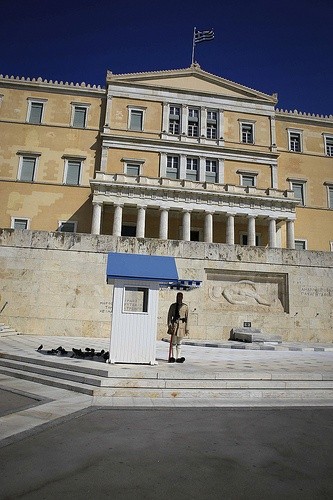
[167,292,190,363]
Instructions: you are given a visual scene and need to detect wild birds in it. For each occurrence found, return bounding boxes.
[38,344,109,362]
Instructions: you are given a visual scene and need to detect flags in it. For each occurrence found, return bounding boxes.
[195,29,216,43]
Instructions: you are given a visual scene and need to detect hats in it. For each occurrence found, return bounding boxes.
[177,293,182,296]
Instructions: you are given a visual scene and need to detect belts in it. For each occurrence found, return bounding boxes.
[173,318,182,320]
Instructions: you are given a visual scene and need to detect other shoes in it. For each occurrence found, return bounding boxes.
[168,358,174,362]
[175,357,184,363]
